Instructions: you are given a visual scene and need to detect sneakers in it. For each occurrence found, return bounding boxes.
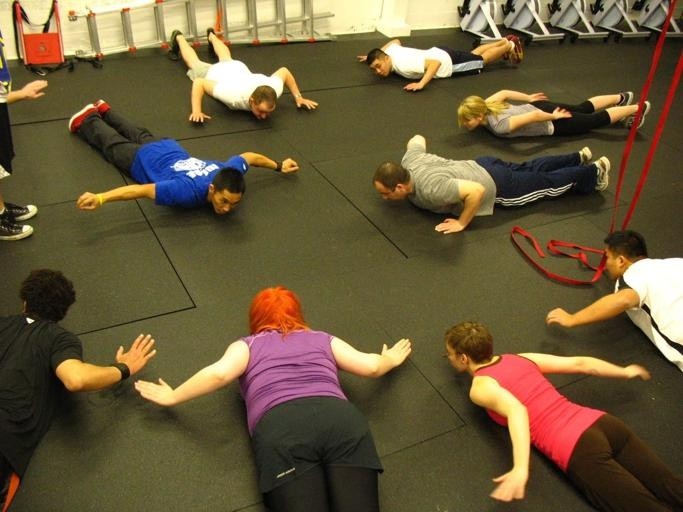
[0,215,34,240]
[69,103,99,133]
[206,28,215,53]
[618,89,632,125]
[95,98,110,112]
[503,34,513,60]
[169,29,183,61]
[3,202,38,221]
[509,36,523,64]
[634,101,651,129]
[580,147,593,164]
[595,156,611,190]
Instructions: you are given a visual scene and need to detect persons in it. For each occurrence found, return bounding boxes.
[136,289,413,512]
[0,31,48,241]
[444,323,682,512]
[169,29,318,125]
[459,89,651,151]
[359,33,524,90]
[545,230,683,373]
[67,98,299,216]
[0,269,156,512]
[375,135,612,234]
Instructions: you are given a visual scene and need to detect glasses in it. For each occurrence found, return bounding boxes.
[444,353,460,360]
